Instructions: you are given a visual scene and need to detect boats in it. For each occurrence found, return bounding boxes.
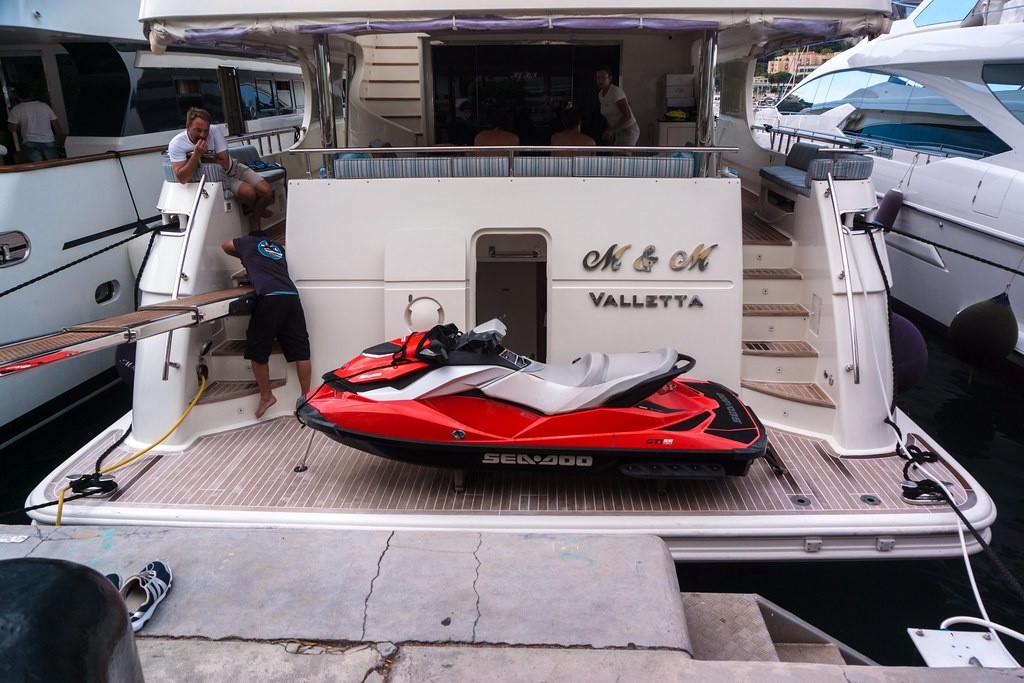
[0,0,352,450]
[742,1,1024,380]
[25,0,1000,563]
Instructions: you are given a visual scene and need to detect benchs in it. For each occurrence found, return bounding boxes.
[333,156,696,178]
[754,141,875,238]
[162,143,288,235]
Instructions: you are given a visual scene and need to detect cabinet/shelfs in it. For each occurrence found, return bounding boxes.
[649,121,696,146]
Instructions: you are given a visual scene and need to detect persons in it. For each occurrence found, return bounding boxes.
[169,109,274,222]
[596,64,640,155]
[551,108,596,157]
[220,231,312,418]
[473,113,518,157]
[8,82,63,161]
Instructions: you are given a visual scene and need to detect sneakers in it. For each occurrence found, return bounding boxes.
[105,570,122,590]
[119,560,174,637]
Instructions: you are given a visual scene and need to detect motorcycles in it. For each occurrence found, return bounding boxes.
[303,312,769,485]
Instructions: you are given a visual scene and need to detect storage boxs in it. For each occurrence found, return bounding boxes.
[657,73,694,86]
[656,86,694,97]
[655,97,696,108]
